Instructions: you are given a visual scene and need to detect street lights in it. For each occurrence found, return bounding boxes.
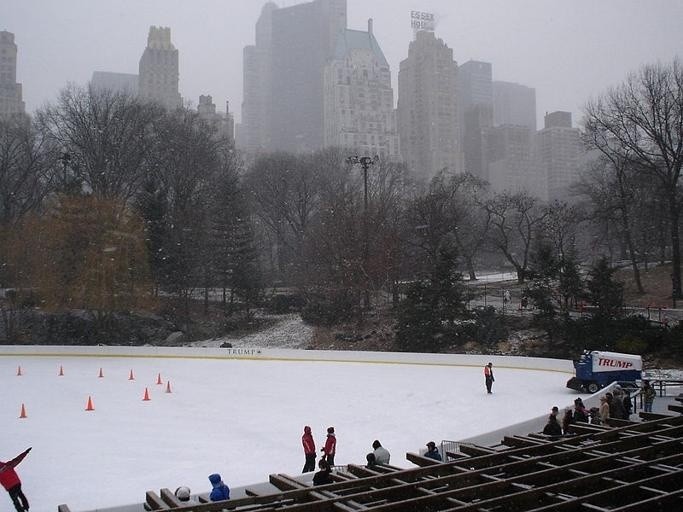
[345,155,379,305]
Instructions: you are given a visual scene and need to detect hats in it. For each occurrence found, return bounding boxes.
[177,487,191,500]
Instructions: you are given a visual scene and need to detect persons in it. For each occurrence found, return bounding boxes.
[0,447,35,511]
[313,459,333,487]
[321,427,336,467]
[302,426,317,473]
[372,440,390,466]
[423,442,441,463]
[366,454,382,469]
[543,385,632,436]
[639,380,656,412]
[208,474,230,502]
[504,291,513,304]
[521,290,529,310]
[175,487,200,506]
[485,362,495,395]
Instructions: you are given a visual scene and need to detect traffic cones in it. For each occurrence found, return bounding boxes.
[20,403,28,418]
[142,388,150,400]
[99,367,104,377]
[17,365,22,376]
[128,369,134,380]
[84,395,95,410]
[58,364,64,376]
[157,372,171,393]
